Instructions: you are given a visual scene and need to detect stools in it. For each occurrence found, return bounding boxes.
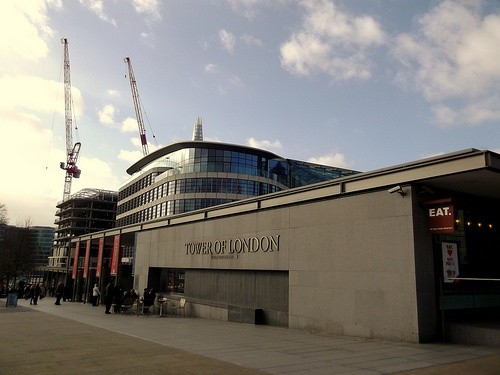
[111,304,116,314]
[121,304,131,316]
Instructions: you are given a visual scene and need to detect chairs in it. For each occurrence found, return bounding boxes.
[171,298,186,317]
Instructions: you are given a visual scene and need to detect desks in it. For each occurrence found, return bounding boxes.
[156,300,166,318]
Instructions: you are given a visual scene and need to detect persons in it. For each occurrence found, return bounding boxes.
[445,261,456,280]
[1,269,157,315]
